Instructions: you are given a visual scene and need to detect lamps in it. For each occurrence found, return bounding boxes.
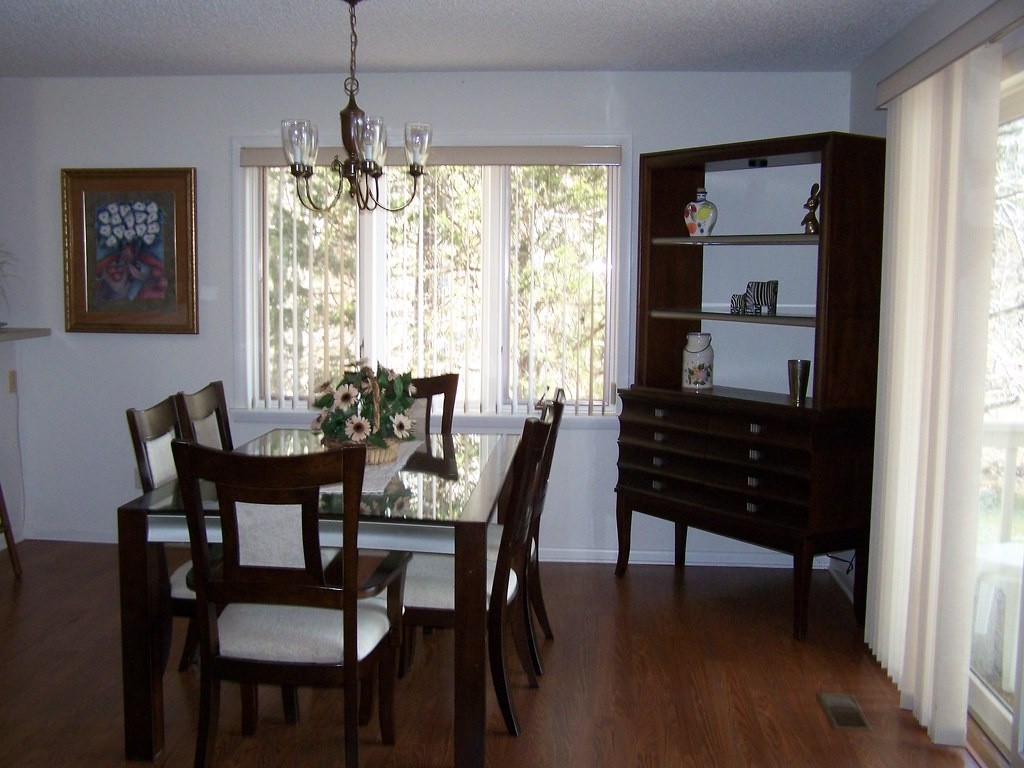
[281,0,433,212]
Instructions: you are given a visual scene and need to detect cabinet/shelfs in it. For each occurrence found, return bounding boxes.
[612,131,887,639]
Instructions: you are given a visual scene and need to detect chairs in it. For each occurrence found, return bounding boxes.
[124,380,300,725]
[361,373,566,737]
[170,437,413,768]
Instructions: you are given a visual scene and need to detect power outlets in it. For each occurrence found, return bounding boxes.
[8,369,18,395]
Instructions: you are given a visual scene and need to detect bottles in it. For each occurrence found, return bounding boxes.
[681,332,714,389]
[684,191,718,236]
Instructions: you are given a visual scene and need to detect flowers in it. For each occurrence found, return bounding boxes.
[319,486,414,519]
[312,356,417,450]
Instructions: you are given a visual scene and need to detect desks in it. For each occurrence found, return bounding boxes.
[117,428,522,768]
[0,327,53,579]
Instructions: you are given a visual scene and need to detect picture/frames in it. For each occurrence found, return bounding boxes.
[60,166,200,336]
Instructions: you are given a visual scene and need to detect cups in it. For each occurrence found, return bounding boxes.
[787,359,811,402]
